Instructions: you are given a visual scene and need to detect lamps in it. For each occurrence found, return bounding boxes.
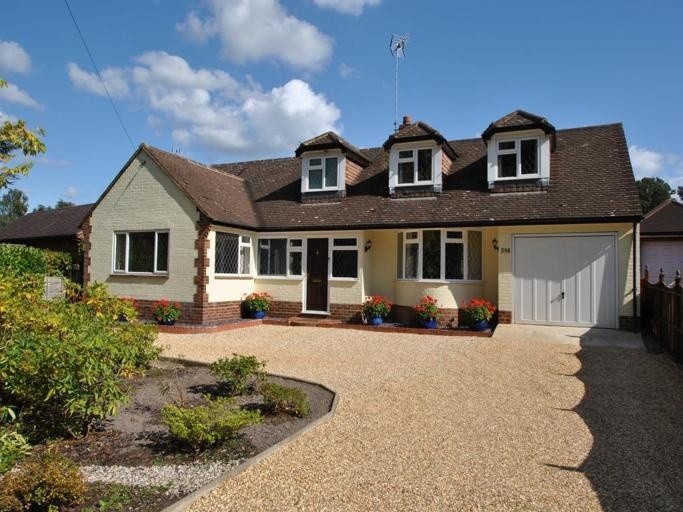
[491,238,499,251]
[364,240,371,252]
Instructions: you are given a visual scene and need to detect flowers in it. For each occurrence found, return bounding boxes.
[243,291,273,312]
[360,295,394,319]
[412,296,444,321]
[151,299,181,322]
[461,296,496,322]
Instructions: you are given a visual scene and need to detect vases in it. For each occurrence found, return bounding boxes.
[366,316,385,325]
[467,319,489,331]
[250,311,265,319]
[423,319,440,331]
[156,319,175,325]
